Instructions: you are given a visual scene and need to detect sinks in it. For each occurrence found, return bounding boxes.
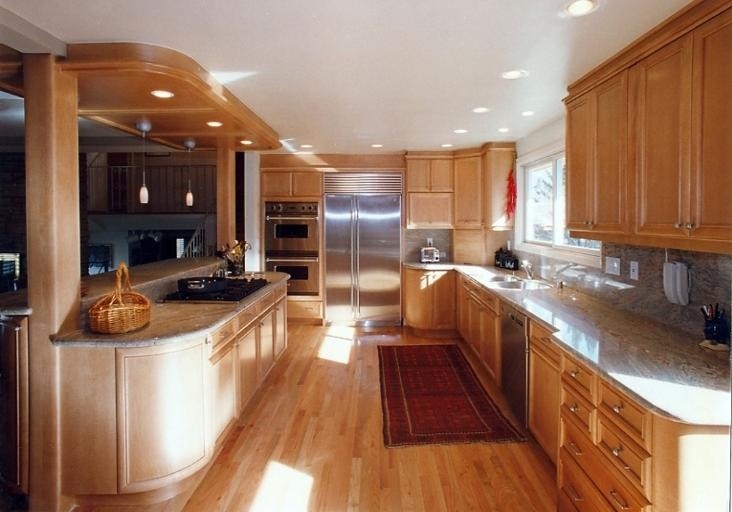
[489,276,519,282]
[498,281,553,290]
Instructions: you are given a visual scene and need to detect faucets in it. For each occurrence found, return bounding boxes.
[521,260,534,279]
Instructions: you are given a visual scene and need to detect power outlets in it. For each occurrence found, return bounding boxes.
[631,261,639,281]
[427,237,433,246]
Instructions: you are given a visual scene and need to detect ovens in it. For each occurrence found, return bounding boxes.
[265,201,318,255]
[266,257,319,295]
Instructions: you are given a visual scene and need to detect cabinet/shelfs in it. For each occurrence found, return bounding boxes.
[58,338,208,494]
[276,279,287,361]
[261,287,276,384]
[238,300,260,419]
[401,263,456,338]
[0,316,29,497]
[457,271,468,344]
[654,408,731,511]
[406,154,454,192]
[627,0,732,256]
[258,167,323,202]
[284,300,325,328]
[525,318,559,468]
[454,151,482,229]
[210,317,237,458]
[482,147,516,232]
[468,279,481,360]
[561,46,626,244]
[481,288,501,389]
[407,193,454,230]
[560,351,653,512]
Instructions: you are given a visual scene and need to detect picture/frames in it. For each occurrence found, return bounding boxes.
[146,152,170,157]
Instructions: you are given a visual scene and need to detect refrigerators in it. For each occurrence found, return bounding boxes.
[324,194,402,327]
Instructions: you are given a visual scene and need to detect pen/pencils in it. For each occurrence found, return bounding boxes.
[700,303,725,320]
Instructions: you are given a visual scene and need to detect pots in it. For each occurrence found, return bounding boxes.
[177,276,226,293]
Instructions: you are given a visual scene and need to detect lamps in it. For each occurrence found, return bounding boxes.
[184,139,196,206]
[135,121,151,204]
[567,0,593,17]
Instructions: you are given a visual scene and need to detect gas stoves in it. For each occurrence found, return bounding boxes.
[164,276,269,302]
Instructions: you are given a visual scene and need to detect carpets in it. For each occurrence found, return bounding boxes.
[379,344,526,449]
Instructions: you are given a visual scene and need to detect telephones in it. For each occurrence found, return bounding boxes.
[662,248,689,307]
